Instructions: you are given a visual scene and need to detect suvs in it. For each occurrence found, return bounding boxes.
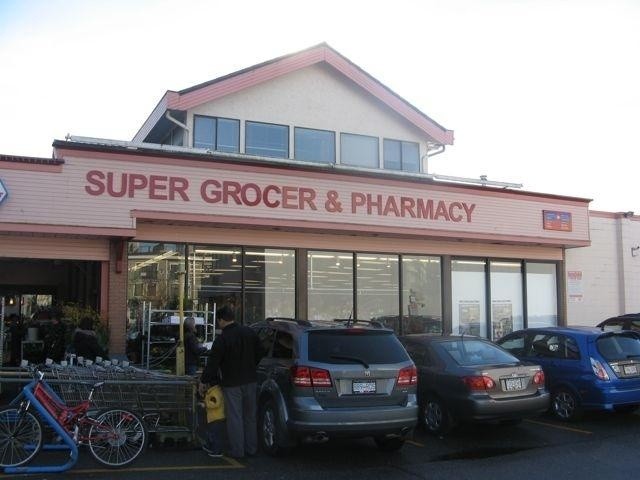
[248,316,420,458]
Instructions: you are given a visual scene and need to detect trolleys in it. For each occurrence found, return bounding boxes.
[21,351,196,448]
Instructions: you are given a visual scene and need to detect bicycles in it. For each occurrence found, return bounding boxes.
[0,363,148,468]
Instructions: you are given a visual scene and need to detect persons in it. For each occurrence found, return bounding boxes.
[198,303,264,461]
[40,313,67,366]
[73,315,109,359]
[184,317,208,376]
[197,375,225,457]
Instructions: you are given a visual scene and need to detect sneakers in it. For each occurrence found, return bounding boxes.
[203,444,224,458]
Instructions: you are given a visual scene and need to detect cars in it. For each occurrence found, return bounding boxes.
[398,331,551,434]
[495,313,640,421]
